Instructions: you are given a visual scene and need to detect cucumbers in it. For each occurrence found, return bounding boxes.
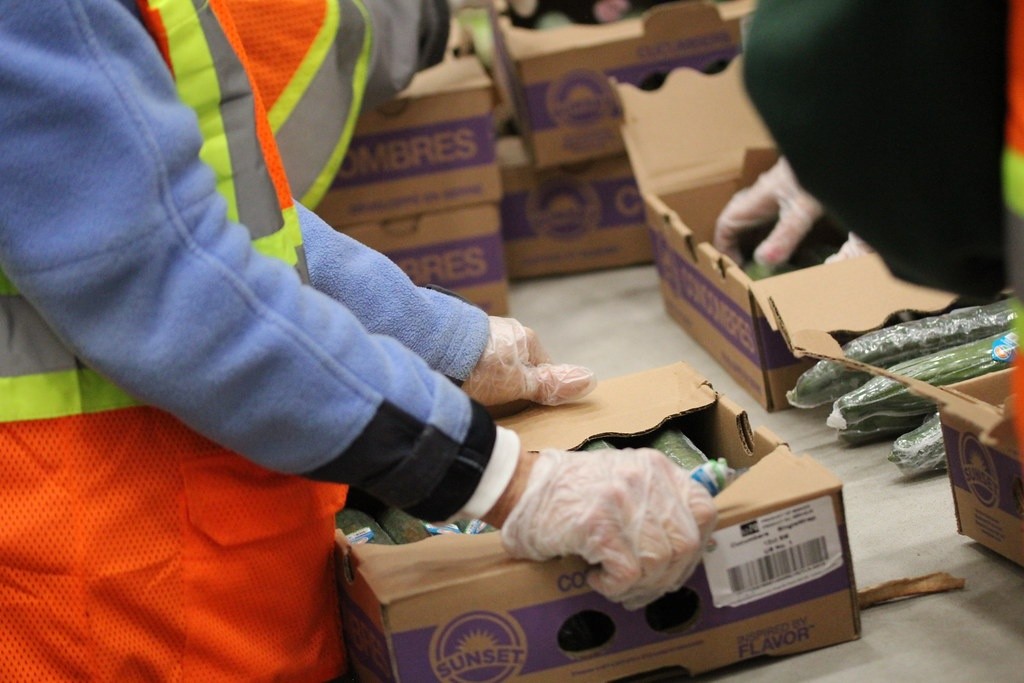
[785,295,1015,474]
[338,420,714,542]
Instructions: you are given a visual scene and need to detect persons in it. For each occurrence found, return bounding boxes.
[0,0,719,683]
[710,152,824,269]
[737,0,1024,474]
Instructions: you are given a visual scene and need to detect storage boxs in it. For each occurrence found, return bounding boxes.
[793,343,1024,566]
[494,136,655,284]
[335,359,863,683]
[616,53,960,416]
[487,1,759,171]
[338,206,510,316]
[314,20,504,228]
